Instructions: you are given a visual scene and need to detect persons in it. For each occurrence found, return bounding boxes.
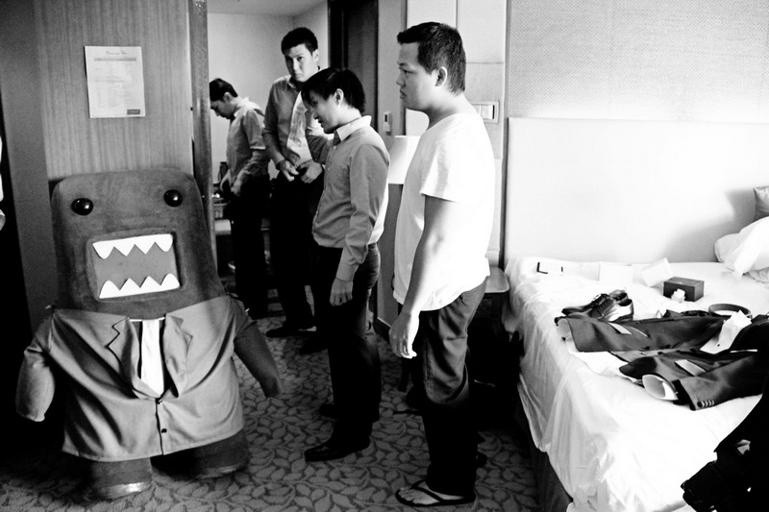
[261,25,338,353]
[389,22,491,505]
[207,77,272,319]
[301,66,391,464]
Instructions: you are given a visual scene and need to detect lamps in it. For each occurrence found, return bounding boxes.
[388,136,421,193]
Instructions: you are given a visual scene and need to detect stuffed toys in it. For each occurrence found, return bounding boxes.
[11,164,283,499]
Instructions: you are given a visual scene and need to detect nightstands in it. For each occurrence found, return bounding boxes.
[390,264,512,410]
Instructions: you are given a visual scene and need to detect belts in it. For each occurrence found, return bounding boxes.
[708,304,752,321]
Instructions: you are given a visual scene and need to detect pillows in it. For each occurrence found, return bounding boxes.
[713,216,769,278]
[752,185,768,222]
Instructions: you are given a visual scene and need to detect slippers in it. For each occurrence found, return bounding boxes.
[426,449,486,476]
[396,479,476,507]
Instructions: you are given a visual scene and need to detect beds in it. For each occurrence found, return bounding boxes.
[505,114,769,511]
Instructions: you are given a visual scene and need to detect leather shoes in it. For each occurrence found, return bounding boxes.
[562,293,628,316]
[319,403,336,419]
[304,437,370,461]
[302,330,329,354]
[266,316,315,337]
[567,298,634,323]
